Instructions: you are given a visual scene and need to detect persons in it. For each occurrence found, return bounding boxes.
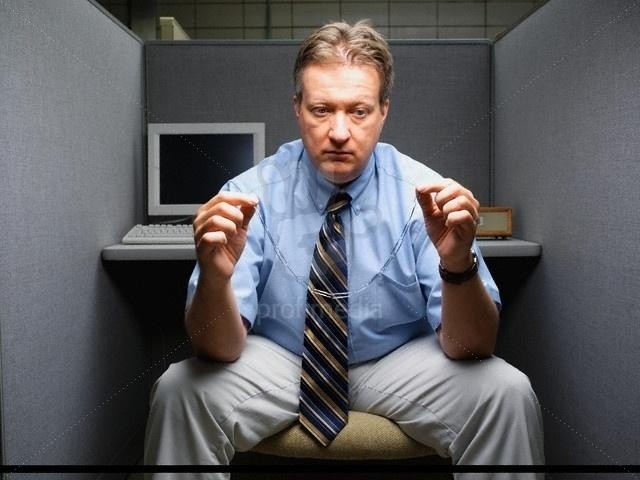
[141,19,547,479]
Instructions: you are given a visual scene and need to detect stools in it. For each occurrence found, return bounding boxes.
[248,410,439,461]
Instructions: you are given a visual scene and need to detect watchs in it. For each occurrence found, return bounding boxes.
[438,250,481,283]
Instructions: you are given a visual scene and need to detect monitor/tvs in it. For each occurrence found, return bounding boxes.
[144,120,266,218]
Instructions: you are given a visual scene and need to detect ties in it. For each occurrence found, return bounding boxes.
[298,192,353,448]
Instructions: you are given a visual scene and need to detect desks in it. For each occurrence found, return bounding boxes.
[102,232,541,260]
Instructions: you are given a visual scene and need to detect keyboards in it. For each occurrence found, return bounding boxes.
[121,223,195,244]
